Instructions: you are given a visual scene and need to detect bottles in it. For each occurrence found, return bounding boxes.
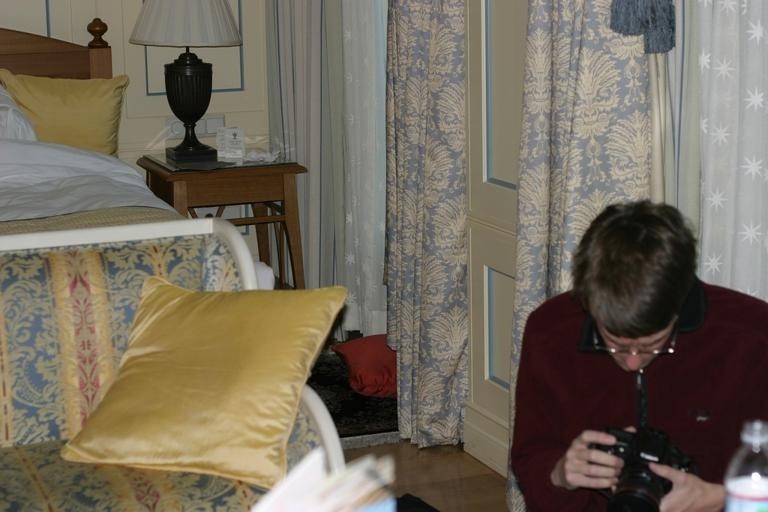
[722,416,768,512]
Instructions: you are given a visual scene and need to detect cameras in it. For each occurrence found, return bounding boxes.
[587,427,702,511]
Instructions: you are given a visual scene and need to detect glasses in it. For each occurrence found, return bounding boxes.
[594,332,676,355]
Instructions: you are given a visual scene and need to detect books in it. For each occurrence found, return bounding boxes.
[250,446,399,511]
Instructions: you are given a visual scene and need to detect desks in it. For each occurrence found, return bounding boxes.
[134,153,309,290]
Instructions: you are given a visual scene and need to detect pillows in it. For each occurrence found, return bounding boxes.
[331,332,398,399]
[0,68,131,159]
[60,275,350,489]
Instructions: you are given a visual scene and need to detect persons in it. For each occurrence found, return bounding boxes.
[509,199,767,512]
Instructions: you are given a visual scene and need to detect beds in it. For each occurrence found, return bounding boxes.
[1,16,276,289]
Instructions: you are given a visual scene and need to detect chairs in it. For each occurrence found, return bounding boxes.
[0,218,347,512]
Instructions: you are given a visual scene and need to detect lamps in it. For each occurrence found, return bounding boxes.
[128,0,244,169]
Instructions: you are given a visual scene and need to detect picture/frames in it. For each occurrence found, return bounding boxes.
[142,0,244,96]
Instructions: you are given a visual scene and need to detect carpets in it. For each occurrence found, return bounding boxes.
[310,338,401,450]
